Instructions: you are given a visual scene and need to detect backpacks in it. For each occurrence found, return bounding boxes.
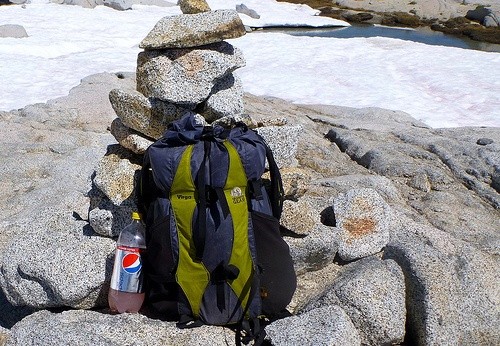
[142,111,310,338]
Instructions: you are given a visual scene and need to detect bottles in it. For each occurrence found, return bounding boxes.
[107,212,147,313]
